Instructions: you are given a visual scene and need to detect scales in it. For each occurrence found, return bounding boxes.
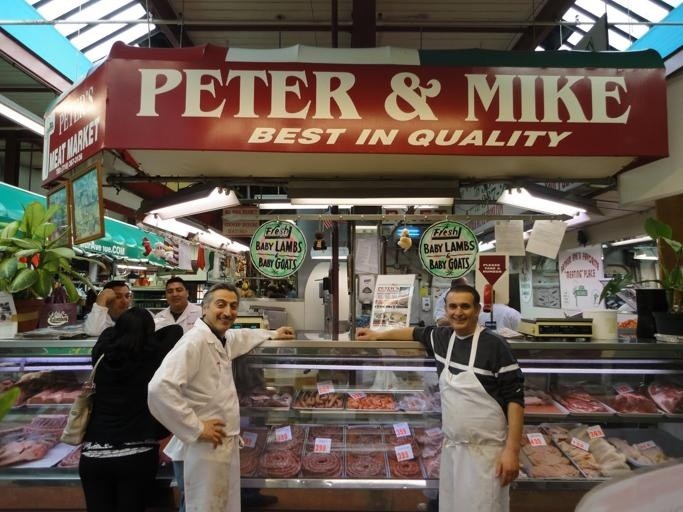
[229,312,270,330]
[515,316,595,342]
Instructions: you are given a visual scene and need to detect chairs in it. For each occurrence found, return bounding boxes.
[573,456,683,512]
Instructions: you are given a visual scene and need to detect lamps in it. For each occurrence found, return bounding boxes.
[139,178,240,220]
[285,180,460,207]
[142,216,252,255]
[313,218,327,250]
[495,178,598,217]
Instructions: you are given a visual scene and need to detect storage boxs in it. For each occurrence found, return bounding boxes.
[221,206,259,238]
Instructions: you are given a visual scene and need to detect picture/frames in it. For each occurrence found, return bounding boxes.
[69,160,106,245]
[46,180,72,250]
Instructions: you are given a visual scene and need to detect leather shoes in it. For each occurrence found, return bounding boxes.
[239,493,278,508]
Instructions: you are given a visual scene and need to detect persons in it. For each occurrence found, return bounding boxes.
[153,276,203,336]
[433,277,469,327]
[85,280,130,337]
[147,283,295,512]
[79,307,184,512]
[355,286,525,512]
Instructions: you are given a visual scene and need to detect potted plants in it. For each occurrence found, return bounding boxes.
[597,218,683,344]
[0,200,109,334]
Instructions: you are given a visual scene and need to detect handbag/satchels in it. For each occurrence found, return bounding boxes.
[40,287,76,328]
[59,379,97,446]
